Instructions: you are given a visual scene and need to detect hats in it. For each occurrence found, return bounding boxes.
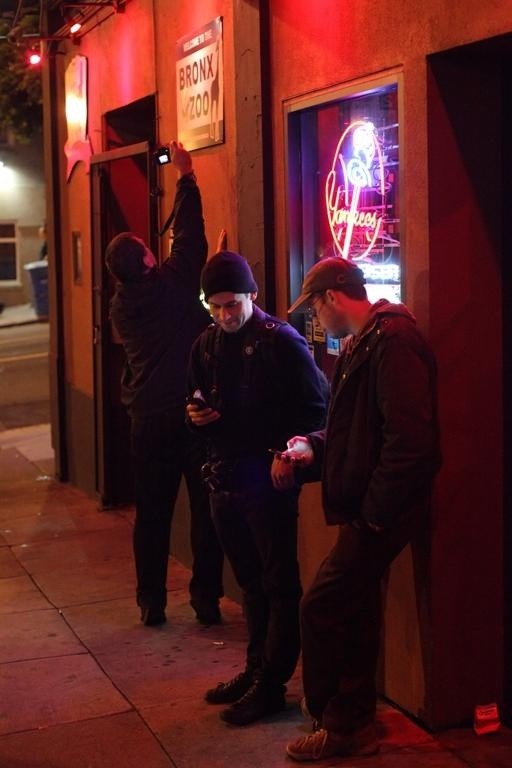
[199,251,258,304]
[287,256,366,314]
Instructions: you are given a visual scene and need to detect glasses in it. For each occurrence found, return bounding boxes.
[304,289,328,320]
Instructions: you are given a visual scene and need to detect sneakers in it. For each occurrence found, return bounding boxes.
[140,604,166,625]
[191,594,221,625]
[286,696,378,761]
[204,670,288,725]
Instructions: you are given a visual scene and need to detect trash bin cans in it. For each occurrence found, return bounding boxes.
[23,260,49,315]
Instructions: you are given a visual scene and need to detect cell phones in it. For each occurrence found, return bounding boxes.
[186,396,209,417]
[268,449,301,463]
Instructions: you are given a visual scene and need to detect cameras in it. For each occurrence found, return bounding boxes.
[153,147,171,166]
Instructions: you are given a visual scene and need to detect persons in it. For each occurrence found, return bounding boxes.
[275,257,442,761]
[104,140,224,626]
[186,251,325,725]
